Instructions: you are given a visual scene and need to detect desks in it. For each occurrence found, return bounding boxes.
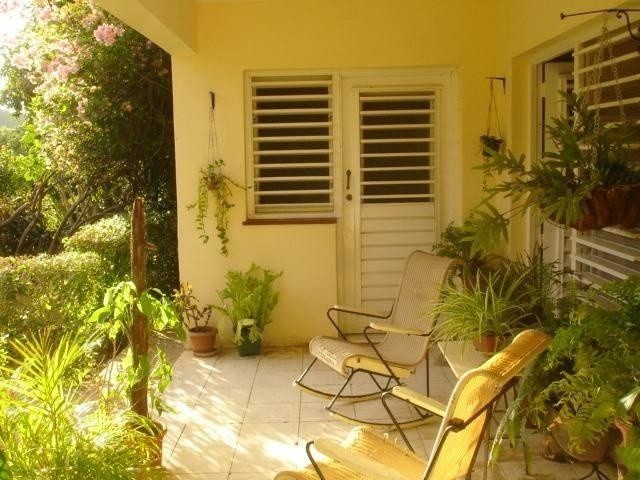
[438,341,525,439]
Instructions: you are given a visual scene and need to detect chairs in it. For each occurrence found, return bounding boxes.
[273,327,551,480]
[293,250,466,430]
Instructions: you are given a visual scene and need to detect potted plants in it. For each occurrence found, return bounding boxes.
[487,274,640,480]
[474,133,502,190]
[88,278,185,470]
[408,255,560,365]
[169,281,218,357]
[208,262,283,357]
[432,221,504,294]
[460,89,640,259]
[185,160,254,258]
